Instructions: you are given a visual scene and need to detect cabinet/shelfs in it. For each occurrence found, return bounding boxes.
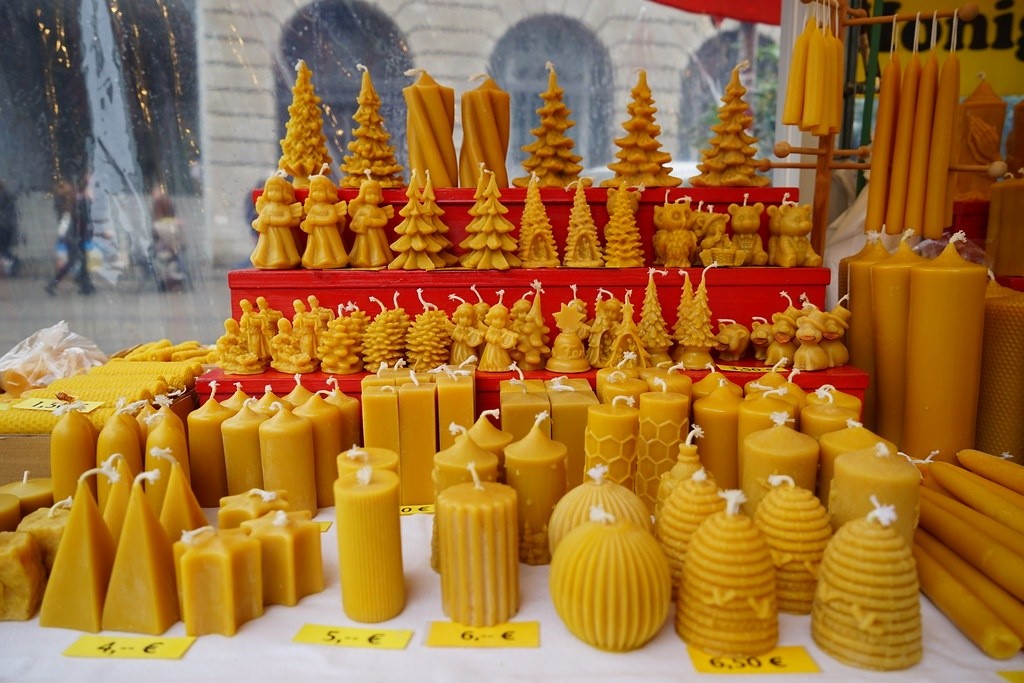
[195,187,870,429]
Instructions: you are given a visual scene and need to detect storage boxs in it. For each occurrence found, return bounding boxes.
[0,389,194,486]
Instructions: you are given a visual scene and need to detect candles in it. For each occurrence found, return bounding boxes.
[0,0,1024,683]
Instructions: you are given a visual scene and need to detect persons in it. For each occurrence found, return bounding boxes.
[147,183,193,295]
[250,175,394,271]
[216,294,335,374]
[445,299,624,373]
[44,179,96,297]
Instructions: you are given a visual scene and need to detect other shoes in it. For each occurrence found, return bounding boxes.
[45,281,58,295]
[80,287,91,295]
